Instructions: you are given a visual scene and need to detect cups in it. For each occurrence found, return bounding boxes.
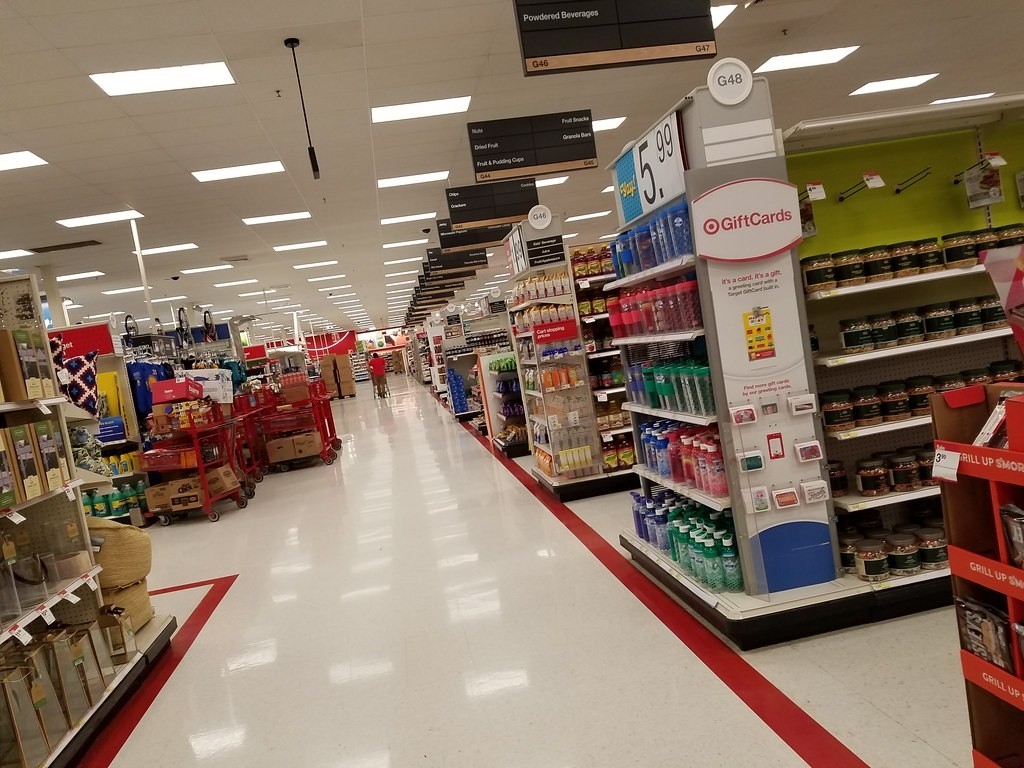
[609,202,694,279]
[606,280,703,338]
[626,361,716,417]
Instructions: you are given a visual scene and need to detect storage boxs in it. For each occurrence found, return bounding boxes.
[0,420,70,510]
[318,353,355,397]
[266,437,296,463]
[169,475,202,494]
[171,491,202,511]
[206,465,241,497]
[281,381,311,403]
[144,483,174,511]
[137,450,198,472]
[153,403,172,427]
[446,363,482,413]
[293,432,322,458]
[0,324,56,402]
[152,376,202,404]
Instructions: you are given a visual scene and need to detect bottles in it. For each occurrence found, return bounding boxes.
[630,489,744,593]
[639,419,729,497]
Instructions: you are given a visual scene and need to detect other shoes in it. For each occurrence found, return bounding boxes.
[380,395,386,398]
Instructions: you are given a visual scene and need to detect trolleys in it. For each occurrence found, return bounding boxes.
[371,373,390,399]
[133,378,341,526]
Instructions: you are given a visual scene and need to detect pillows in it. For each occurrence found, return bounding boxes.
[67,425,114,476]
[65,348,100,417]
[51,332,72,403]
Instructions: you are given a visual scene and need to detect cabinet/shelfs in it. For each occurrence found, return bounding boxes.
[145,404,251,524]
[379,336,433,381]
[488,371,528,452]
[230,388,270,481]
[575,274,636,477]
[261,379,338,465]
[0,275,179,768]
[929,383,1024,768]
[508,294,597,454]
[99,439,147,520]
[602,254,733,512]
[352,353,370,381]
[434,344,446,389]
[807,263,1014,592]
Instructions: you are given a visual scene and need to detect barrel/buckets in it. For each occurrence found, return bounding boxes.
[578,286,619,354]
[594,398,631,431]
[101,444,140,476]
[600,434,635,473]
[568,243,616,279]
[588,356,626,390]
[80,480,149,516]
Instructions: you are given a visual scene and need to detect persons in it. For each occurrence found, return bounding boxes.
[367,353,387,398]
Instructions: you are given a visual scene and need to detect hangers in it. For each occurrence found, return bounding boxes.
[126,345,170,367]
